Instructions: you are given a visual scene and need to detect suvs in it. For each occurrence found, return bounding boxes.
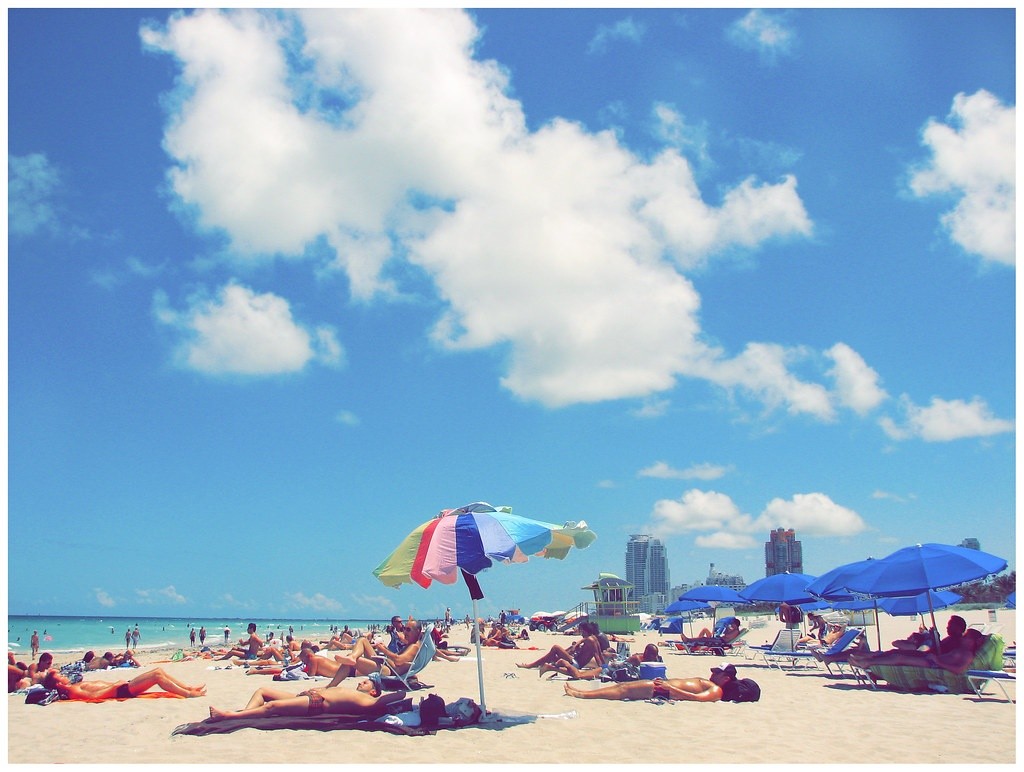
[529,615,560,632]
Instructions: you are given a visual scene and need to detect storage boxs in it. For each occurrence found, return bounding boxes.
[639,661,667,680]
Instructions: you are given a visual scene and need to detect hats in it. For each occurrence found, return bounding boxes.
[710,662,737,674]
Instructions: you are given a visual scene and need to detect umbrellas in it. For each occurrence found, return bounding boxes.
[678,584,757,655]
[371,501,599,719]
[775,599,831,635]
[832,591,962,649]
[739,570,854,666]
[664,599,711,638]
[1002,591,1016,609]
[845,542,1007,656]
[804,556,921,652]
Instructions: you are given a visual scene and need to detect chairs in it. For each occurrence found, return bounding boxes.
[368,622,442,691]
[751,624,1016,705]
[665,628,750,656]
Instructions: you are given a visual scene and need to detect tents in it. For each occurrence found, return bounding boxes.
[657,618,684,635]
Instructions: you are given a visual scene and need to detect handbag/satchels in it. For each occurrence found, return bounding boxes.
[616,642,630,658]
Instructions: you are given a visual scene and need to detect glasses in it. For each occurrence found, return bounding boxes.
[394,620,403,624]
[402,627,417,632]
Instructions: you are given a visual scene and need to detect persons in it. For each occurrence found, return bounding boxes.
[43,670,208,703]
[515,619,635,668]
[563,663,737,702]
[810,632,922,666]
[539,642,663,679]
[209,679,406,721]
[681,616,740,642]
[8,607,522,691]
[807,623,846,644]
[798,633,815,643]
[327,620,421,688]
[848,616,983,674]
[779,601,799,629]
[807,613,827,644]
